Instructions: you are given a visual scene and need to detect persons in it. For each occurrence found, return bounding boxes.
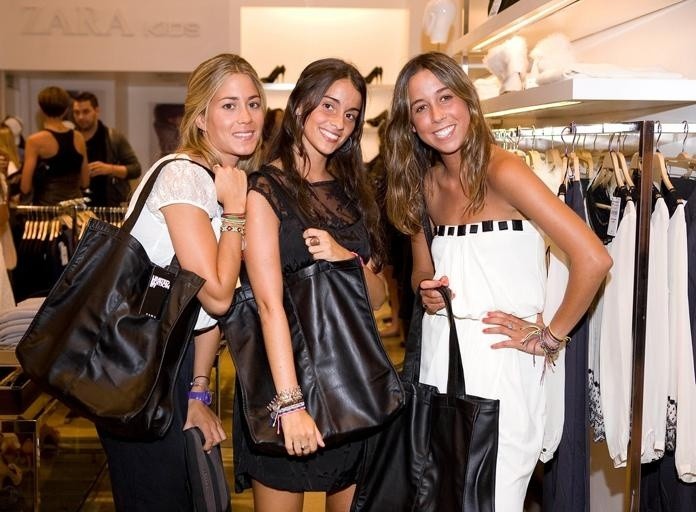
[0,113,27,148]
[380,52,616,512]
[224,56,393,512]
[0,123,31,213]
[88,52,271,512]
[72,90,142,221]
[18,86,90,222]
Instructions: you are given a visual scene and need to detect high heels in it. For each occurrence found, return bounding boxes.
[258,64,286,82]
[361,65,382,84]
[366,110,389,125]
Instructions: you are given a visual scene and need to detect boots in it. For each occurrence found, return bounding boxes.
[481,34,529,95]
[529,30,578,86]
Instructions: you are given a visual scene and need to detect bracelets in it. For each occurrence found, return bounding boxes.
[189,375,208,390]
[217,209,247,238]
[534,324,566,359]
[267,386,307,419]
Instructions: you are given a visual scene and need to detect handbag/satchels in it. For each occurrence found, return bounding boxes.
[348,279,500,511]
[110,176,133,202]
[213,169,409,450]
[13,157,224,447]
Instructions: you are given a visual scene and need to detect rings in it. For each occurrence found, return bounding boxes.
[506,321,514,330]
[422,303,427,309]
[212,165,220,171]
[300,444,309,450]
[310,237,320,247]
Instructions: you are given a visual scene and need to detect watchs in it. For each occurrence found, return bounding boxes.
[184,388,214,405]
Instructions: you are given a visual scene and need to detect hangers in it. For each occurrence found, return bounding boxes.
[11,196,127,243]
[481,121,696,220]
